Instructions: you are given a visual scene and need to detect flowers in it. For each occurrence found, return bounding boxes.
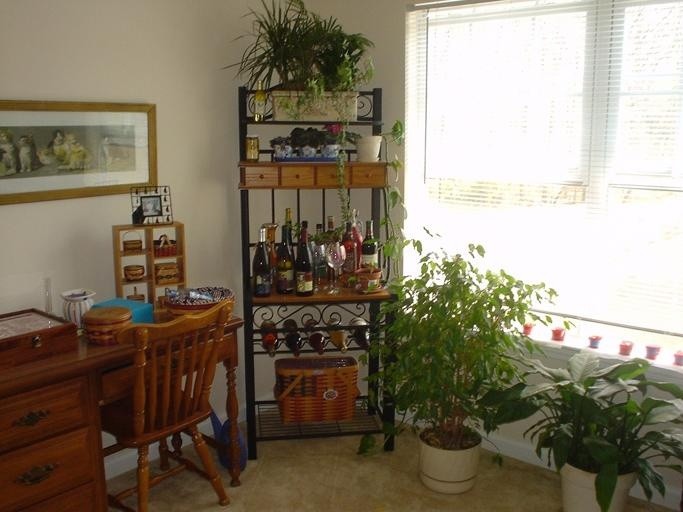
[321,124,342,145]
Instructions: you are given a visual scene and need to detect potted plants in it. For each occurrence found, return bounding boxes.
[219,0,376,121]
[288,127,324,158]
[356,226,576,495]
[269,136,292,158]
[475,347,682,511]
[354,119,407,283]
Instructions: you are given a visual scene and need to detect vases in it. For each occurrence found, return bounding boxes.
[319,145,340,158]
[358,135,383,164]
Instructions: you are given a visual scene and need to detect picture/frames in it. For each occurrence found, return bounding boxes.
[0,99,157,207]
[129,186,173,226]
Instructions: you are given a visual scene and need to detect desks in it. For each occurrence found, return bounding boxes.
[0,317,244,512]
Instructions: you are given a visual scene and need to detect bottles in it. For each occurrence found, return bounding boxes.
[277,225,295,294]
[326,318,348,353]
[296,221,314,297]
[326,216,343,277]
[305,319,326,355]
[256,80,266,122]
[283,319,302,358]
[260,320,279,357]
[351,208,362,270]
[247,135,259,162]
[253,226,270,297]
[264,223,278,289]
[349,317,371,351]
[341,222,357,288]
[362,220,378,268]
[312,224,329,294]
[285,208,295,264]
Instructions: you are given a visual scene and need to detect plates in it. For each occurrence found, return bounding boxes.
[355,157,379,163]
[272,156,341,162]
[354,284,383,294]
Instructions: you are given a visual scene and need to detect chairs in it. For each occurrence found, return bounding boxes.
[96,298,235,512]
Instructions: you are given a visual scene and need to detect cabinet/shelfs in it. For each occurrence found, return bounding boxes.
[236,86,399,461]
[111,220,187,313]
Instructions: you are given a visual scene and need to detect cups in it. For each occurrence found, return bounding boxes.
[325,242,346,295]
